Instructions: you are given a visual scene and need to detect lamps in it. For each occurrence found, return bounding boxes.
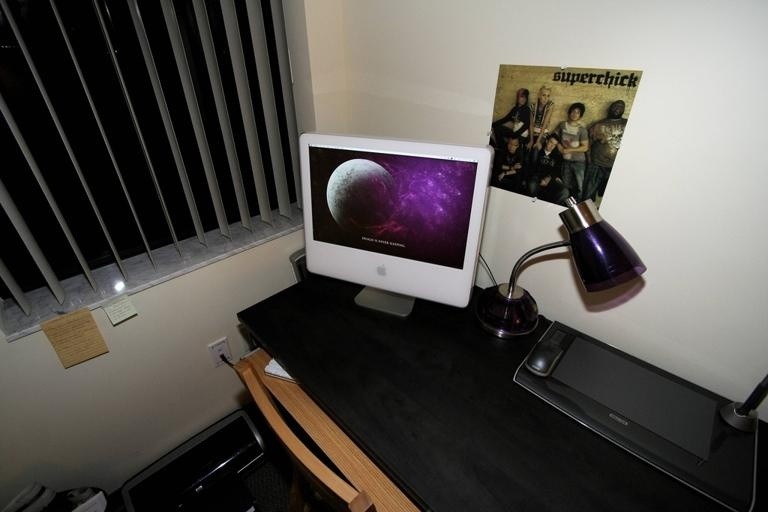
[475,196,645,339]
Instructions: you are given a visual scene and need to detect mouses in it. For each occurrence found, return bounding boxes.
[525,340,565,377]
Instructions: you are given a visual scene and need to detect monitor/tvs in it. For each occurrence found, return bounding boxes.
[298,132,495,316]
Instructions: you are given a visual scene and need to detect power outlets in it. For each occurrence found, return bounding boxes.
[208,336,232,368]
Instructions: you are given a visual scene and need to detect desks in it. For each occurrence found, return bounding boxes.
[236,280,768,512]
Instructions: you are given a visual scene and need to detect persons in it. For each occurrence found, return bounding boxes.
[491,81,628,211]
